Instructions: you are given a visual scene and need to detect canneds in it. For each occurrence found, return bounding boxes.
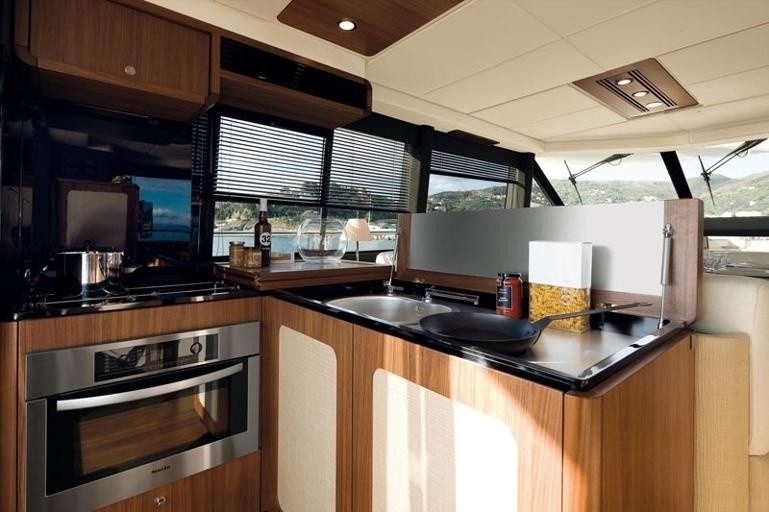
[229,241,246,269]
[496,271,524,319]
[244,245,262,268]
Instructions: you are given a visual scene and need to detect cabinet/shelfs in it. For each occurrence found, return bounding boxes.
[261,296,352,512]
[220,29,373,129]
[351,324,695,512]
[13,1,217,122]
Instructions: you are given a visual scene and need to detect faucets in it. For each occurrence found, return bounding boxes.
[385,230,401,297]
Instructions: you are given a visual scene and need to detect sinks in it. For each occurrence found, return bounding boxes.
[329,297,449,324]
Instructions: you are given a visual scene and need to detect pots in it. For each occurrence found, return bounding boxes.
[53,250,126,292]
[420,302,653,357]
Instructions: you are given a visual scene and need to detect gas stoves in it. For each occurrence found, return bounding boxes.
[16,255,240,322]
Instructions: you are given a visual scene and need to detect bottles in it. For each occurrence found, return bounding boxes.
[254,199,272,267]
